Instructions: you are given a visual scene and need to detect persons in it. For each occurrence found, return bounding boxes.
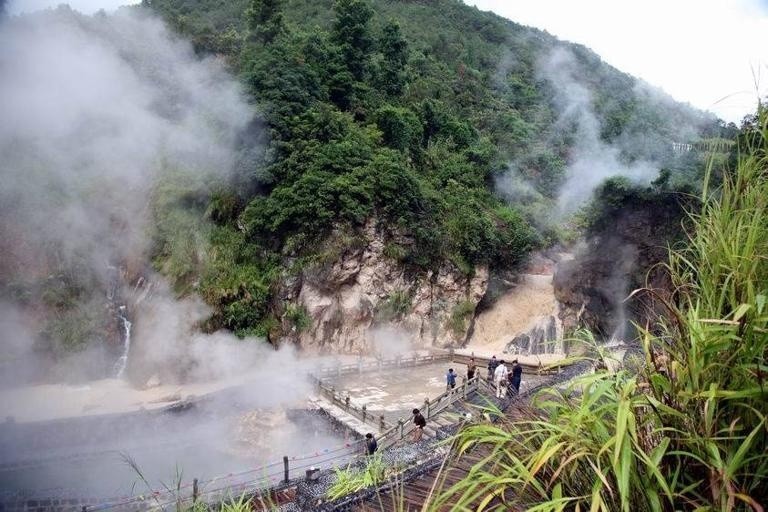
[493,360,511,401]
[487,355,499,380]
[509,359,522,396]
[363,433,378,464]
[411,408,426,443]
[447,367,458,396]
[465,356,476,385]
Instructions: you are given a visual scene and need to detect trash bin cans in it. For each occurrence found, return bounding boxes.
[305,468,320,482]
